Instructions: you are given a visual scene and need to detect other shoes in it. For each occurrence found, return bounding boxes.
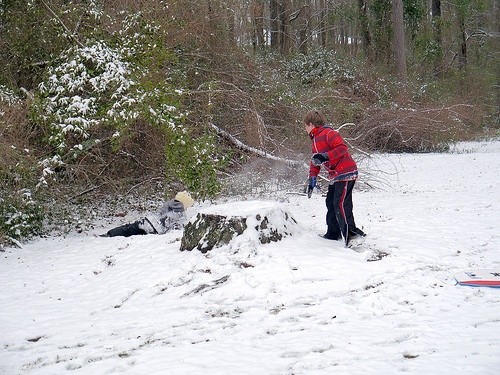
[319,233,343,241]
[345,233,367,250]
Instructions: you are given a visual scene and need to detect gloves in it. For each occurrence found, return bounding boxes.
[306,177,316,198]
[310,152,329,168]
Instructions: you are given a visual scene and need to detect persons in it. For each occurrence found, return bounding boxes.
[302,108,368,250]
[96,190,195,238]
